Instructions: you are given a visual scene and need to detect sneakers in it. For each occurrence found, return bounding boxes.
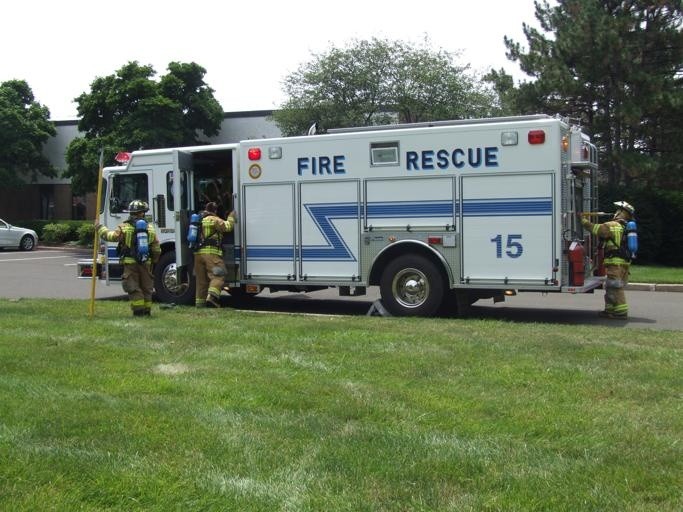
[599,311,628,319]
[133,307,151,316]
[206,293,221,308]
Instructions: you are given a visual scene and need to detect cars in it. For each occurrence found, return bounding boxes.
[0,219,39,252]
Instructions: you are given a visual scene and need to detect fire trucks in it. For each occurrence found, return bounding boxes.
[75,106,616,320]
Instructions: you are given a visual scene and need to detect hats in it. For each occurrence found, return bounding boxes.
[614,200,636,215]
[122,200,150,214]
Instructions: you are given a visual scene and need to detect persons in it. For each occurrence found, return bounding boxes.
[94,199,162,316]
[577,200,636,320]
[192,201,235,308]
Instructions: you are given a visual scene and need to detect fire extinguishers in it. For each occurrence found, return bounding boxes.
[593,245,607,276]
[563,229,587,286]
